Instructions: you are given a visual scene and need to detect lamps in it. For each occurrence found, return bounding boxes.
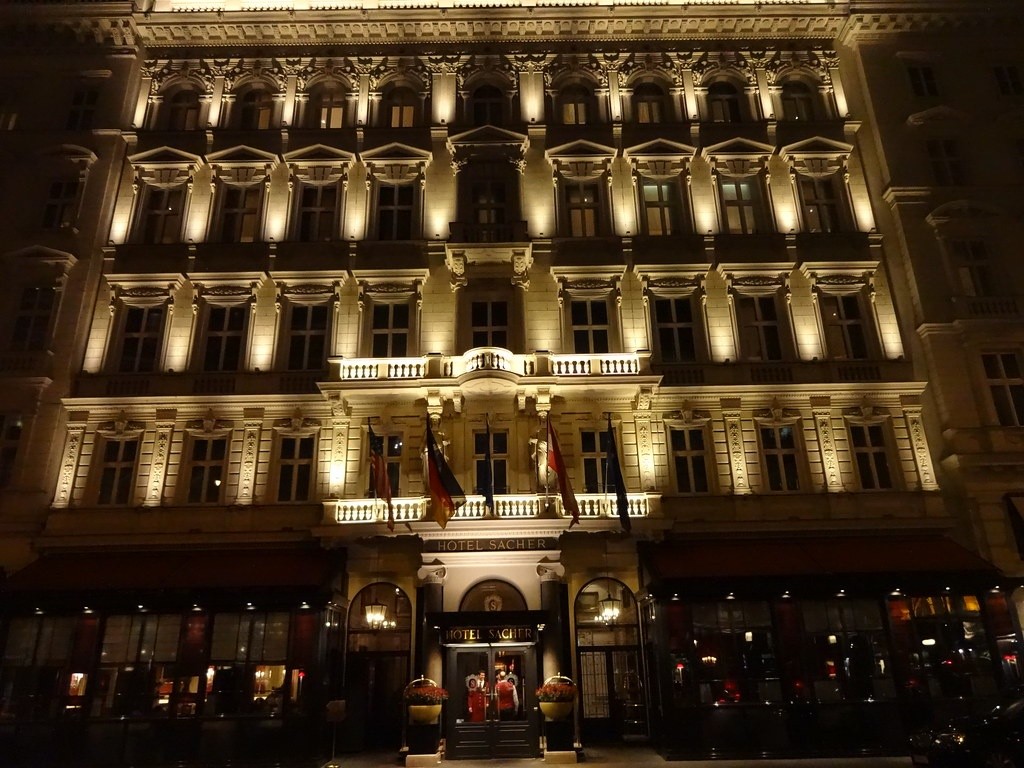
[598,594,651,632]
[347,597,388,637]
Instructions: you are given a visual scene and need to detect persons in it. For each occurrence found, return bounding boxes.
[494,672,520,721]
[467,671,487,723]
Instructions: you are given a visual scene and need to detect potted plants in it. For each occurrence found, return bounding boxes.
[535,683,579,721]
[402,686,449,723]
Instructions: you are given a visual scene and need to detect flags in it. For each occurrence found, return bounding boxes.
[367,426,395,532]
[606,417,632,535]
[481,420,496,519]
[427,418,465,528]
[545,411,579,530]
